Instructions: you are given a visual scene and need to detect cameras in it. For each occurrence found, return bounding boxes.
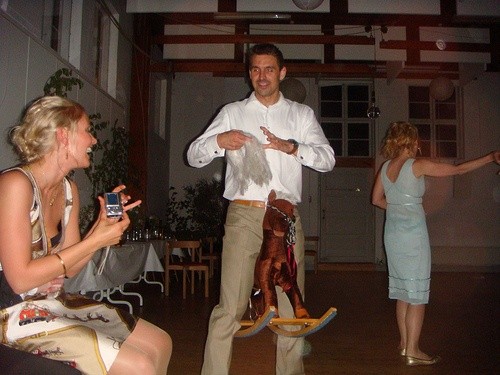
[104,192,123,218]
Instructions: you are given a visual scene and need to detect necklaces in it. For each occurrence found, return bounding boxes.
[49,198,54,206]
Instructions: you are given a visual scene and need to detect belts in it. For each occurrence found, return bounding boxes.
[233,199,296,210]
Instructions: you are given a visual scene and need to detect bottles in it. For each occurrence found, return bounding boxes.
[124,228,175,243]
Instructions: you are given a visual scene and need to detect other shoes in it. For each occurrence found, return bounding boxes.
[399,346,407,356]
[407,356,443,366]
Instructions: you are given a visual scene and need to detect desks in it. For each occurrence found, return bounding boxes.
[64,239,164,316]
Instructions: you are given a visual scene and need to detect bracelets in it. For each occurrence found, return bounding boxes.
[55,253,66,274]
[287,139,298,155]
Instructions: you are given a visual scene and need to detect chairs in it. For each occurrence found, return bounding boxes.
[165,240,209,297]
[200,237,224,279]
[304,236,320,274]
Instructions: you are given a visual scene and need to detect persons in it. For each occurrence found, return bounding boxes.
[0,96,172,375]
[371,122,500,366]
[188,42,336,375]
